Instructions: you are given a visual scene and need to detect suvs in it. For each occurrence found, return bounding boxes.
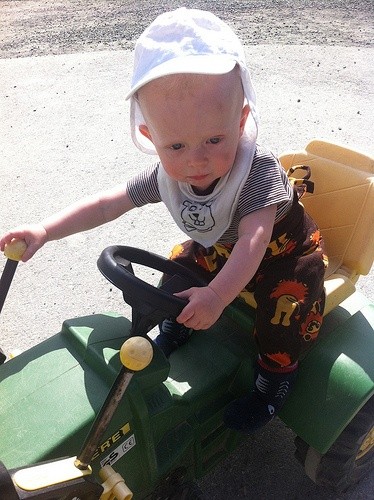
[1,140,373,500]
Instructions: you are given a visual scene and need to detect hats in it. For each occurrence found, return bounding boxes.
[125,7,259,156]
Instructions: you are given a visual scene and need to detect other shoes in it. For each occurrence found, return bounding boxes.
[153,330,189,356]
[223,385,287,431]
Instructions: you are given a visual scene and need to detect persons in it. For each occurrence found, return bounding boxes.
[0,7,328,430]
